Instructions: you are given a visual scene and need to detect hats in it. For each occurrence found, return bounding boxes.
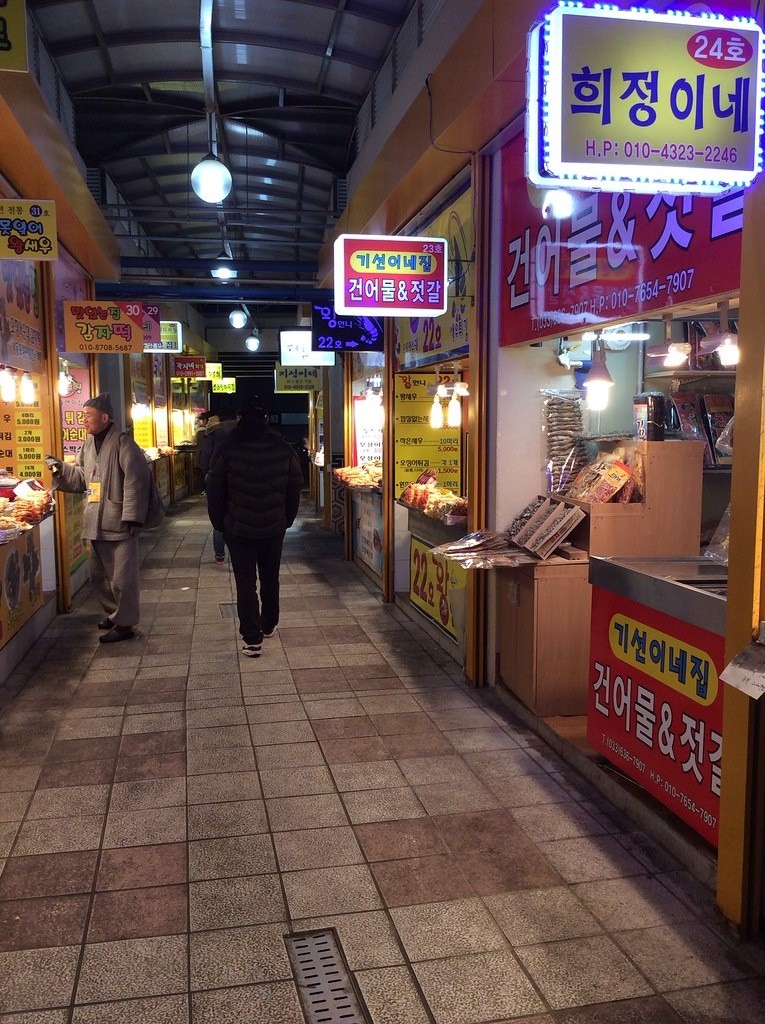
[83,392,113,418]
[195,413,207,419]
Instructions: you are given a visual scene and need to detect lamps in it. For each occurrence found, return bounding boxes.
[0,363,34,406]
[190,98,232,203]
[647,313,692,368]
[695,301,740,366]
[228,303,259,352]
[210,221,238,286]
[582,329,615,410]
[428,360,468,429]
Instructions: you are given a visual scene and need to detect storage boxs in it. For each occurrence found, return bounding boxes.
[502,495,585,560]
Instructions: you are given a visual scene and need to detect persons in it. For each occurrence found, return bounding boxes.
[195,409,240,565]
[44,392,150,643]
[207,398,302,656]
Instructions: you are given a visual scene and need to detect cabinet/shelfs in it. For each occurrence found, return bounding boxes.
[642,370,736,474]
[549,440,705,556]
[495,557,591,717]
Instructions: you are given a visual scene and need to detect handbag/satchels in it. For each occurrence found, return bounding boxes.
[119,432,164,529]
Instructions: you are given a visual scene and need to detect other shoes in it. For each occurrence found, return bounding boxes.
[99,625,135,643]
[215,553,225,565]
[263,624,278,637]
[242,644,261,656]
[98,619,114,629]
